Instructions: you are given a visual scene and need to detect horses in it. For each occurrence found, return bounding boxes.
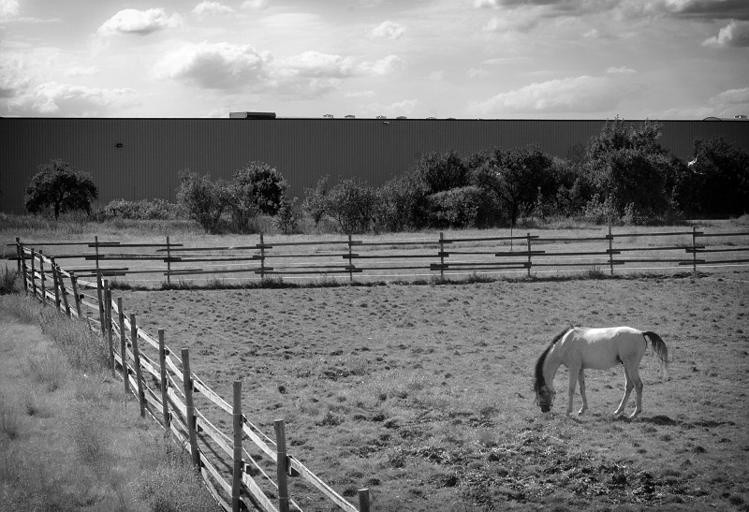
[533,324,668,419]
